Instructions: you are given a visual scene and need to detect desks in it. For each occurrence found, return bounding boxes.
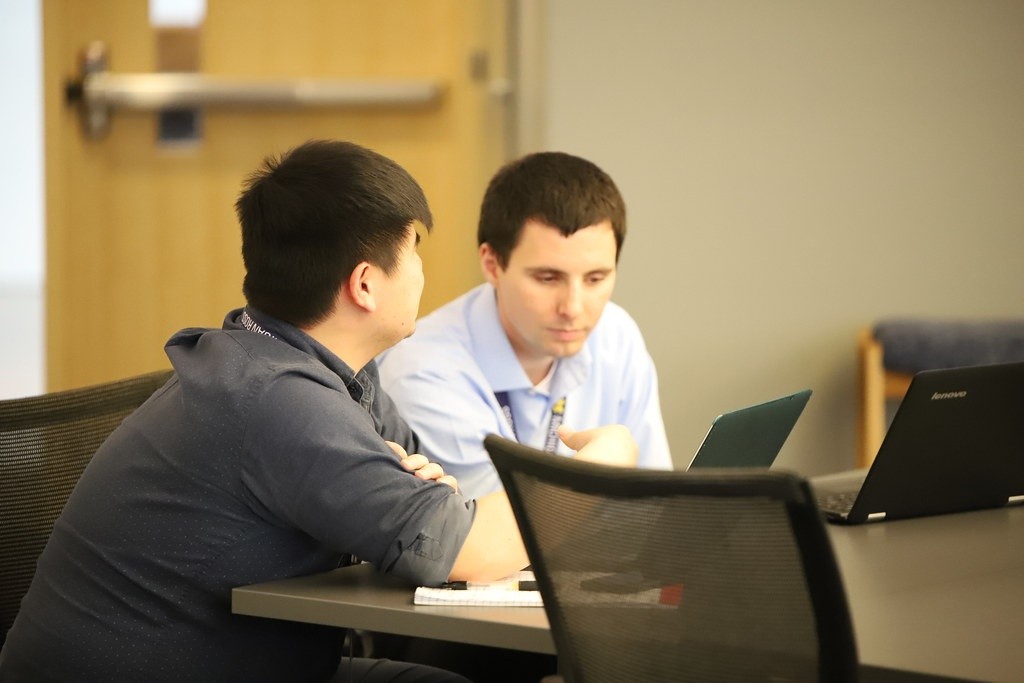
[233,454,1024,683]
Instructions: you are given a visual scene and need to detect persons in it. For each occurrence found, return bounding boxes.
[371,151,674,501]
[0,138,641,683]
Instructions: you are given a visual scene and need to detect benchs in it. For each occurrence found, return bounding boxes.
[856,318,1024,471]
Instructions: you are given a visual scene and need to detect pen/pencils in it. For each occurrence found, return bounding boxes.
[442,580,540,592]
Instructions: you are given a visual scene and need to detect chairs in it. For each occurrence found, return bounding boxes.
[483,433,861,683]
[0,369,179,650]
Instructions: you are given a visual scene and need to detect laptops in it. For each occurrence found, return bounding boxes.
[550,387,814,572]
[808,360,1024,525]
